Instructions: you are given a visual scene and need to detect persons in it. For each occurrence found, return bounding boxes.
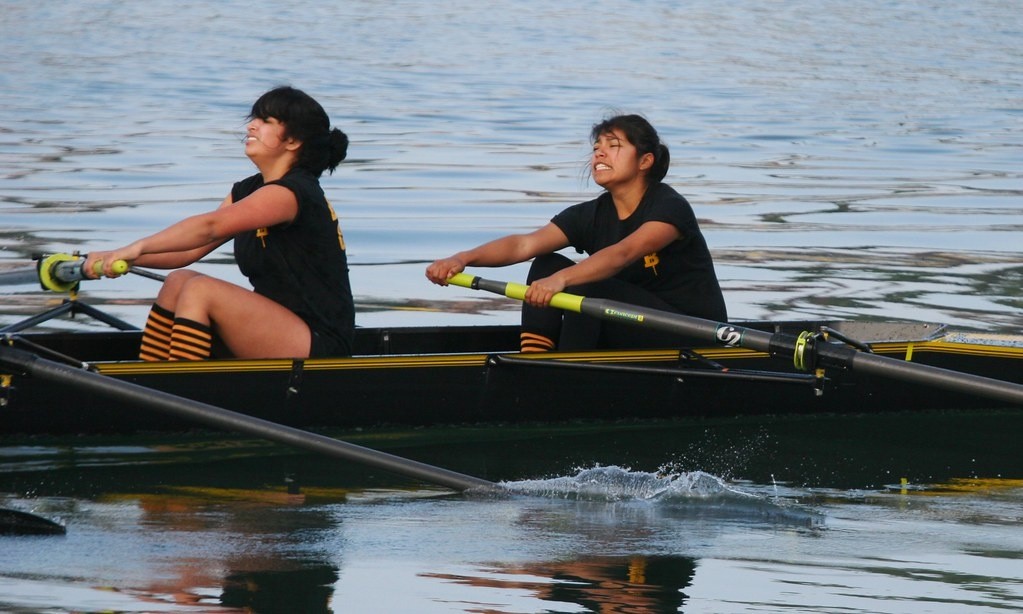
[80,86,357,363]
[425,113,730,353]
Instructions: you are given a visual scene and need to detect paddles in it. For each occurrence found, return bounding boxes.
[0,263,128,288]
[447,274,1019,402]
[0,347,509,496]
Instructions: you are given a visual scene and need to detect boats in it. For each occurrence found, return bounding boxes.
[1,318,1023,488]
